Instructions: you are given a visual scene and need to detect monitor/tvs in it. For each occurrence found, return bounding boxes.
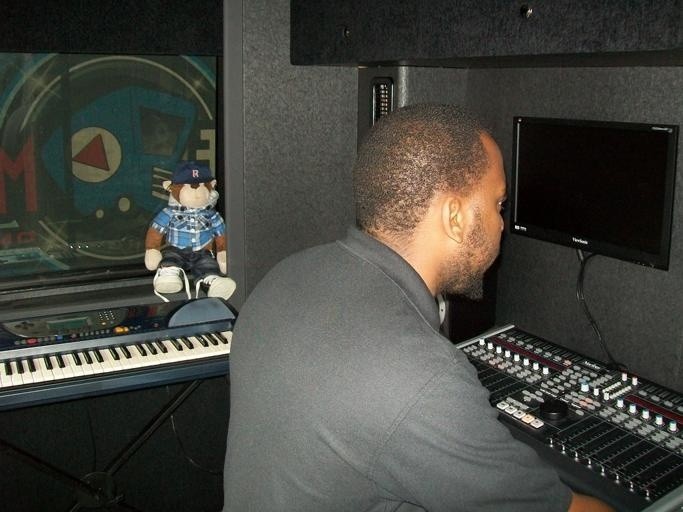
[508,115,679,271]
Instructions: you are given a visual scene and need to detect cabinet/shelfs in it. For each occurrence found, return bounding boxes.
[287,0,683,67]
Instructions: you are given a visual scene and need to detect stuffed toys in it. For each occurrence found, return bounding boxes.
[145,161,236,302]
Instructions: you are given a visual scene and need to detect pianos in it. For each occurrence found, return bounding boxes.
[0,279,239,412]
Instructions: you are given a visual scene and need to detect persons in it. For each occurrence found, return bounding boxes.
[222,103,608,511]
